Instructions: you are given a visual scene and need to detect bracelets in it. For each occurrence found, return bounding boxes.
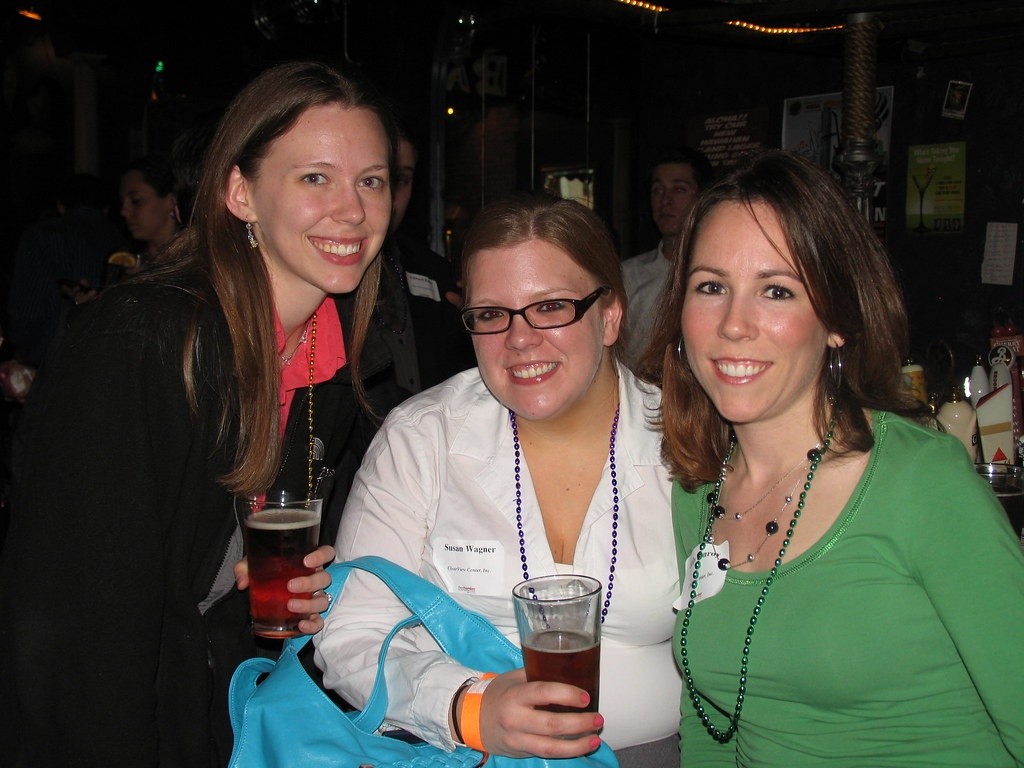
[451,672,498,753]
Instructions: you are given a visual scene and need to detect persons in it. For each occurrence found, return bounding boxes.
[0,61,465,768]
[619,140,720,391]
[643,147,1024,767]
[308,186,685,768]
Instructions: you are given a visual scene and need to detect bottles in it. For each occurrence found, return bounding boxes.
[918,392,944,433]
[935,384,979,464]
[898,359,927,429]
[969,353,990,419]
[990,362,1012,393]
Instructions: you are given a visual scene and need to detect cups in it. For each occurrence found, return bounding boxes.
[974,463,1024,543]
[234,487,324,638]
[513,574,602,738]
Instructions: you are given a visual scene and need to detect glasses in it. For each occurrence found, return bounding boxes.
[462,287,604,335]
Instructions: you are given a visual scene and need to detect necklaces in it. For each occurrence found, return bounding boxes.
[257,307,318,509]
[680,409,851,745]
[505,408,620,638]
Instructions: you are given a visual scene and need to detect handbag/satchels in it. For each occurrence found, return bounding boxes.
[228,555,618,768]
